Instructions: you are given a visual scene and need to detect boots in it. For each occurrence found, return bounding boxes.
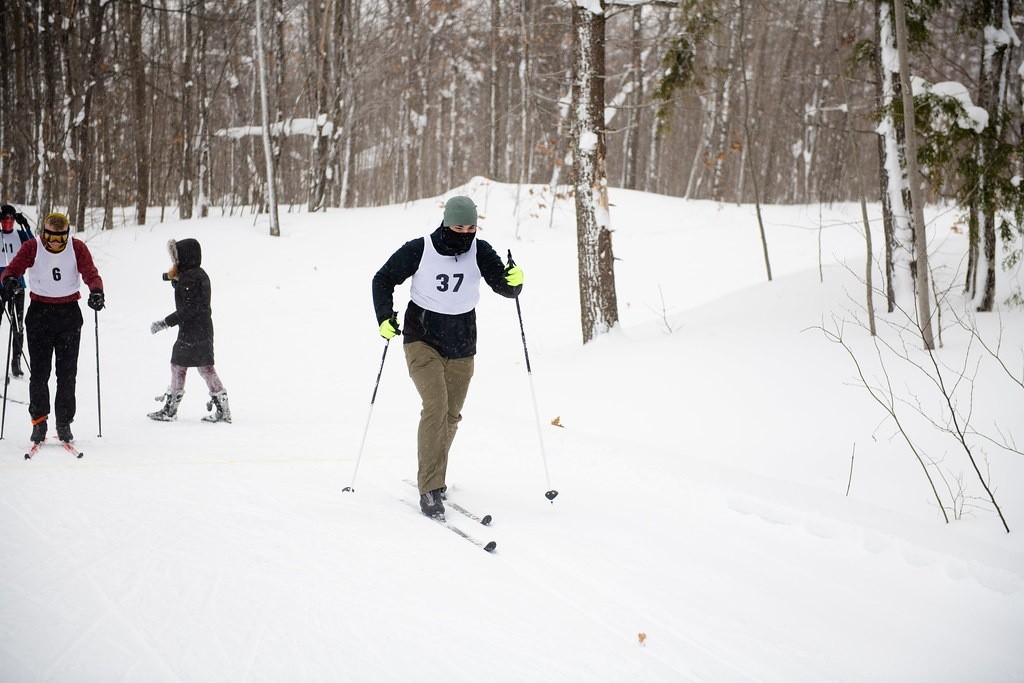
[147,386,185,421]
[201,388,232,423]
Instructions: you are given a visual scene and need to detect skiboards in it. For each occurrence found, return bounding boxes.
[21,435,84,461]
[341,473,499,553]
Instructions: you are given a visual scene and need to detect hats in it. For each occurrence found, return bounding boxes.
[443,196,477,227]
[0,205,16,222]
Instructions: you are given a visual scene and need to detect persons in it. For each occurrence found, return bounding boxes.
[371,196,523,518]
[0,214,104,444]
[146,238,232,423]
[0,204,35,376]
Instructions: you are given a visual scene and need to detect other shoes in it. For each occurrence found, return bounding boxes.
[11,357,24,376]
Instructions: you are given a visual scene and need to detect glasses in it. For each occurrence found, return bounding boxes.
[44,229,68,243]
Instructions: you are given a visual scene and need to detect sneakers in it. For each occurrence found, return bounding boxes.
[420,488,447,514]
[56,418,74,441]
[31,414,48,441]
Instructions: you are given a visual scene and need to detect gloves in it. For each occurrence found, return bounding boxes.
[88,288,105,310]
[4,276,19,296]
[14,213,27,225]
[150,320,166,334]
[503,260,523,290]
[379,316,401,339]
